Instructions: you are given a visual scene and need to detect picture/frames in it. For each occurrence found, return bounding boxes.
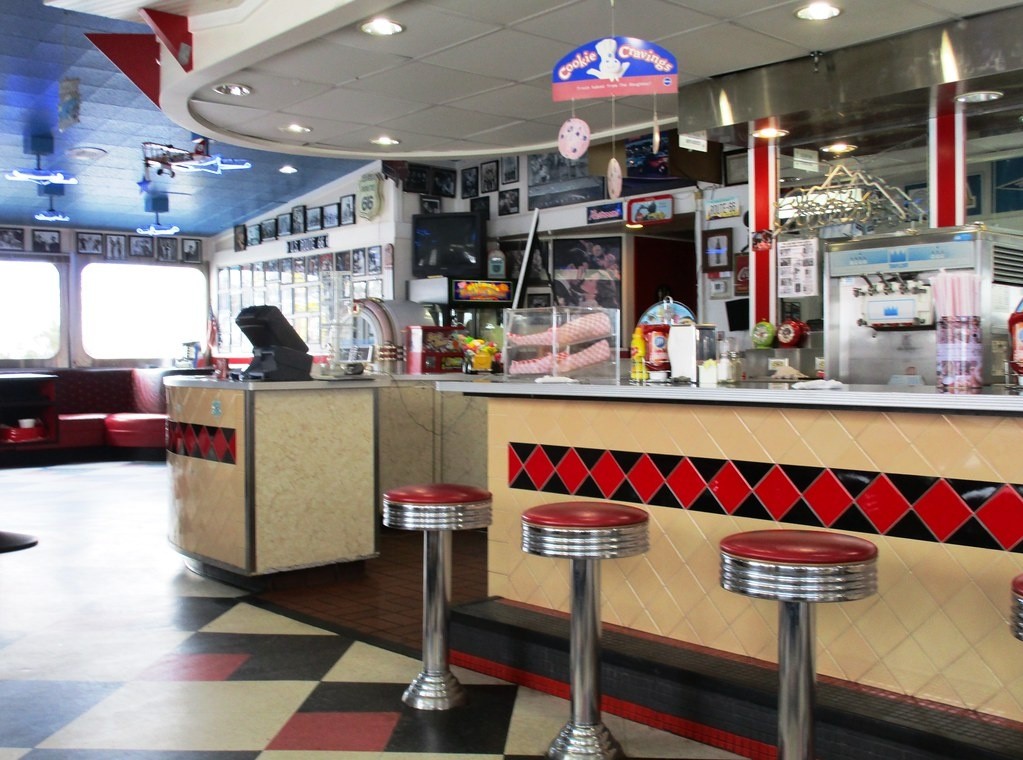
[77,233,104,254]
[233,194,356,252]
[216,245,383,353]
[156,237,178,262]
[702,227,732,272]
[128,235,153,257]
[104,233,126,261]
[626,194,674,226]
[496,236,622,348]
[461,168,478,198]
[497,189,520,216]
[480,157,519,194]
[470,196,490,221]
[181,238,202,264]
[0,227,24,251]
[402,163,457,214]
[31,229,61,253]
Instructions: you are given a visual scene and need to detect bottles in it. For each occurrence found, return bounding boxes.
[718,352,747,383]
[630,326,648,380]
[487,242,506,280]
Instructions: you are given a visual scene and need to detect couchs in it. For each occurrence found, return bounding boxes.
[0,368,215,453]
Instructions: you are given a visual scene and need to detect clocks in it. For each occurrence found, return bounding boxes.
[752,319,775,349]
[775,318,801,348]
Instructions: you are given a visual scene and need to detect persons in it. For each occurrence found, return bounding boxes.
[571,270,620,333]
[552,248,591,328]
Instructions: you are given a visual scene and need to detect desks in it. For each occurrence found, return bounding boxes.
[0,372,60,553]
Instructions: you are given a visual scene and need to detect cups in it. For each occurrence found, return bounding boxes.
[935,316,984,389]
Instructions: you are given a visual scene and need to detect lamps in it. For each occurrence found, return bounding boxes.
[136,193,180,235]
[5,133,79,186]
[34,181,70,222]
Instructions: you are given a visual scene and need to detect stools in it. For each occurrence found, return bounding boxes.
[520,501,650,760]
[382,484,492,711]
[718,529,880,759]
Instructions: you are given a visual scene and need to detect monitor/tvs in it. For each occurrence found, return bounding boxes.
[236,305,308,354]
[412,212,488,281]
